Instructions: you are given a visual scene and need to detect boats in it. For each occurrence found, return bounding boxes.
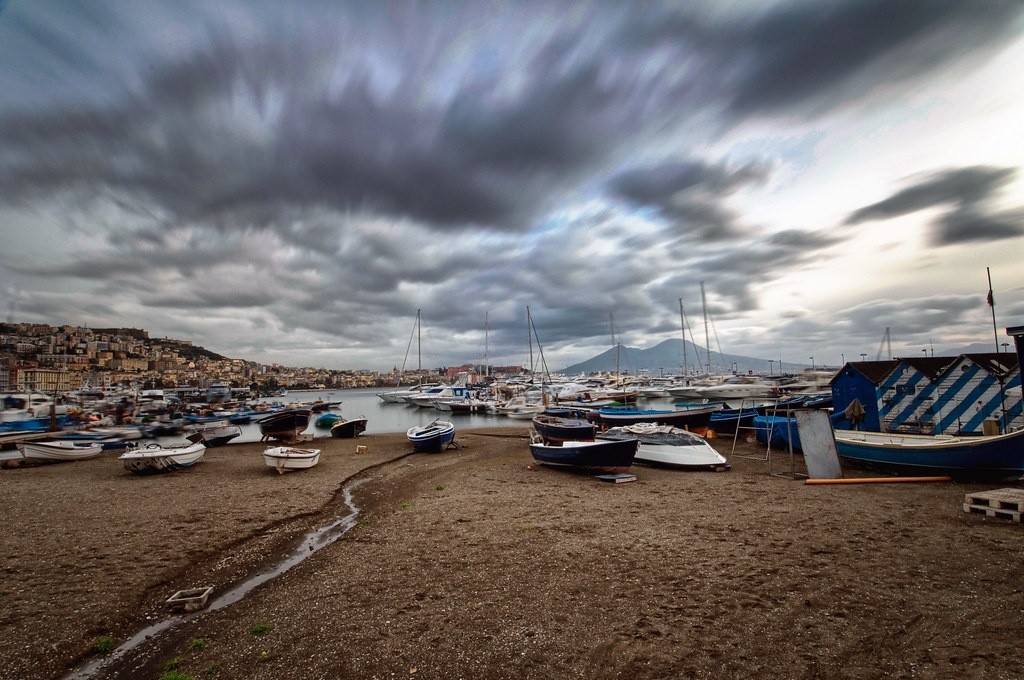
[532,413,599,446]
[596,426,727,469]
[262,444,321,475]
[406,418,455,453]
[528,439,642,474]
[16,439,106,463]
[119,441,206,473]
[376,264,1024,484]
[0,370,369,468]
